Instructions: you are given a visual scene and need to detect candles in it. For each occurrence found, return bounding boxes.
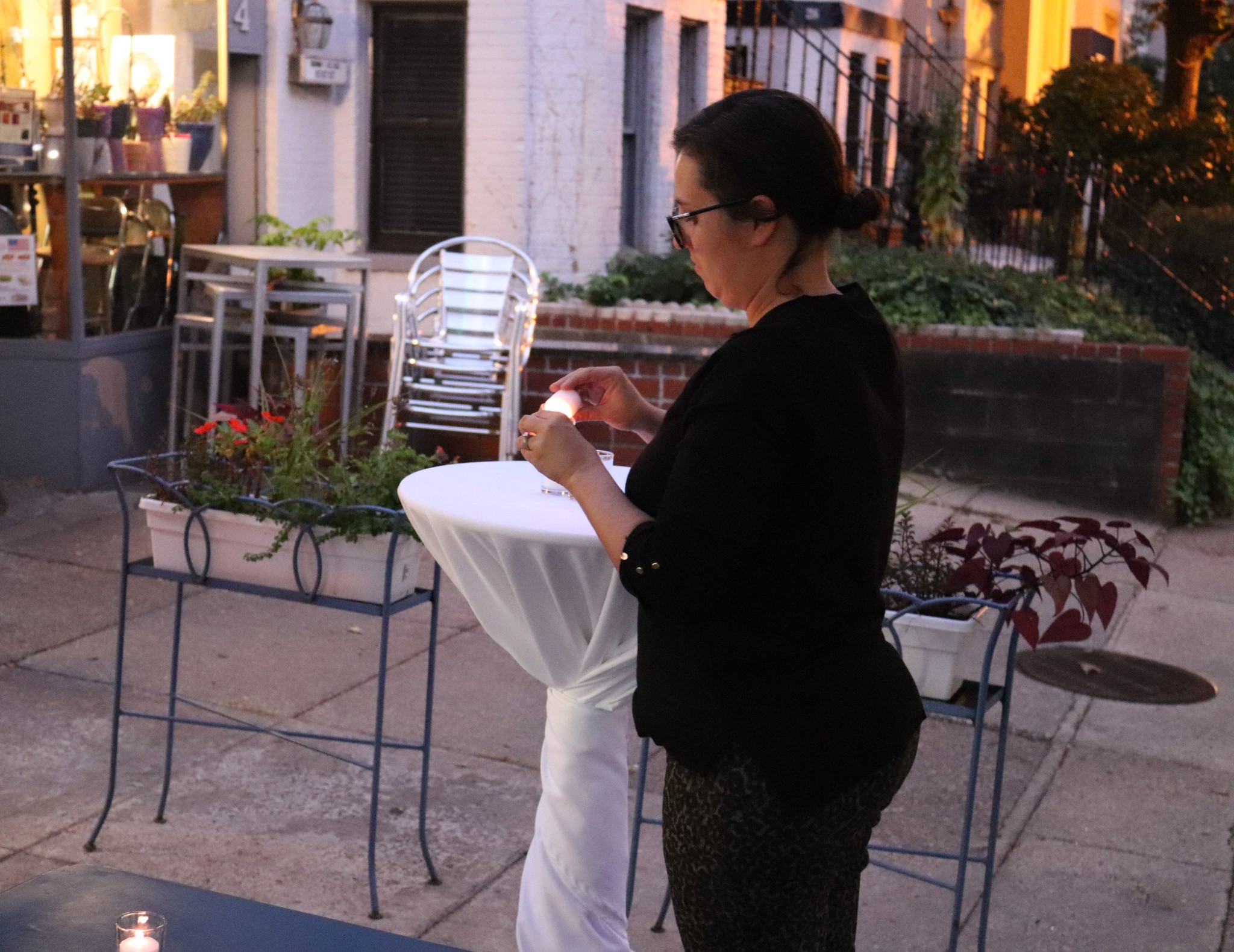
[115,911,167,952]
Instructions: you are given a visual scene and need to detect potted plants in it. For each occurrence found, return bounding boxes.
[882,506,1170,700]
[80,84,112,136]
[247,214,366,316]
[173,71,227,172]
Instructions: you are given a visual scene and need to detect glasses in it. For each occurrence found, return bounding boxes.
[664,196,750,249]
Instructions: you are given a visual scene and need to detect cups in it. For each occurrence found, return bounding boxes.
[115,911,169,952]
[541,449,615,501]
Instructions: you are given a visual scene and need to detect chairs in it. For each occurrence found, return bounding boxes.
[33,195,175,332]
[379,236,540,458]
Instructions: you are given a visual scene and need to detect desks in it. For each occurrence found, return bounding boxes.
[166,244,371,478]
[395,462,639,952]
[0,862,457,952]
[0,173,230,335]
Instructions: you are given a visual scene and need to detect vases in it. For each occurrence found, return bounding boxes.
[139,498,422,605]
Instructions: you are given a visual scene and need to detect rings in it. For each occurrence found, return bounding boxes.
[522,432,534,450]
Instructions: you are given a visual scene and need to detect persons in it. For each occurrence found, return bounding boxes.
[523,88,928,951]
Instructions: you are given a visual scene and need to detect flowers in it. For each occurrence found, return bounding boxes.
[137,313,459,541]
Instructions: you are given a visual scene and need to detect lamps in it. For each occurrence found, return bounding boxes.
[292,0,333,54]
